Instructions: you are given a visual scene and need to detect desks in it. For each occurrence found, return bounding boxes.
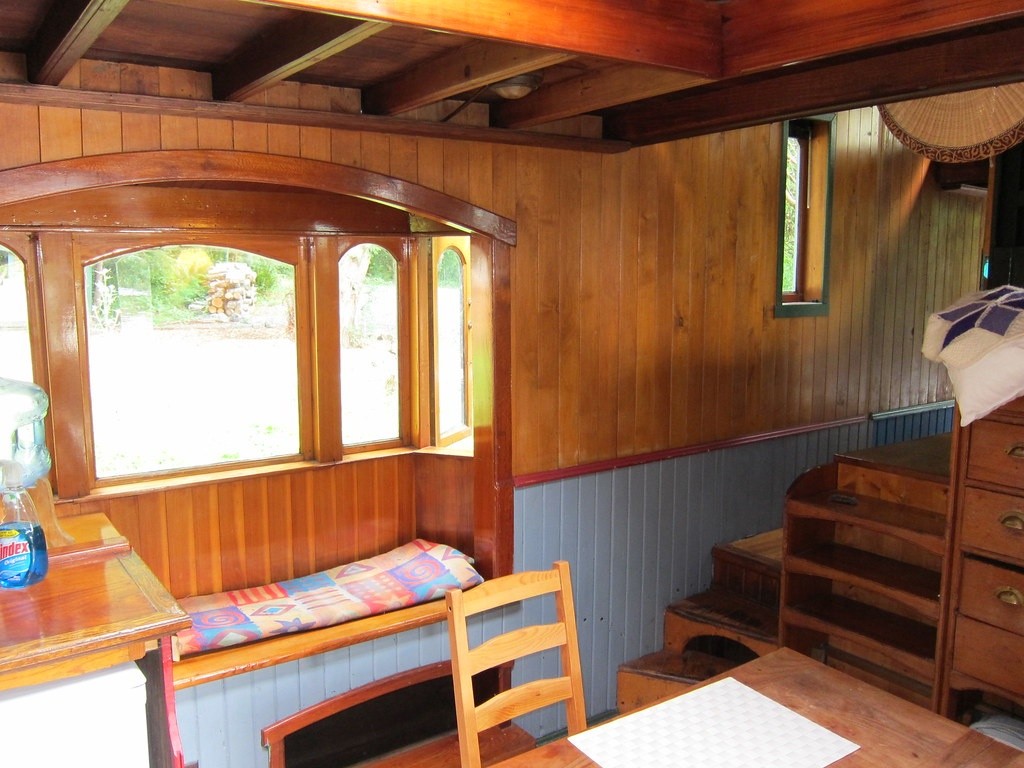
[483,646,987,767]
[0,511,196,768]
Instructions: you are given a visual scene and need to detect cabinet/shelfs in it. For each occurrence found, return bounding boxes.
[929,399,1024,725]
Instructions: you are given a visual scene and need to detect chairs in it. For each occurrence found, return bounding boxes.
[444,558,588,768]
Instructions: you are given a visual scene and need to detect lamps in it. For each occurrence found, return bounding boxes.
[487,74,543,100]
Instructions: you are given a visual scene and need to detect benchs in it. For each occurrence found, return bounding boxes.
[104,455,507,768]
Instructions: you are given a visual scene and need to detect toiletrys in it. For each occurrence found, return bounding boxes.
[0,458,50,590]
